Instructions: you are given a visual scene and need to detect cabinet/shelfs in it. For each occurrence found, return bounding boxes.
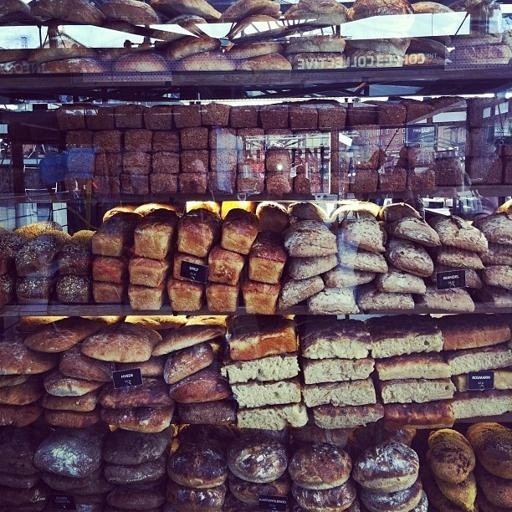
[1,68,512,455]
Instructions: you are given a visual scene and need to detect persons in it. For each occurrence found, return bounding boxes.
[178,145,310,193]
[118,171,141,197]
[352,135,512,191]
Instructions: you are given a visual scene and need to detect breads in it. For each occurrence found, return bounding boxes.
[0,0,512,512]
